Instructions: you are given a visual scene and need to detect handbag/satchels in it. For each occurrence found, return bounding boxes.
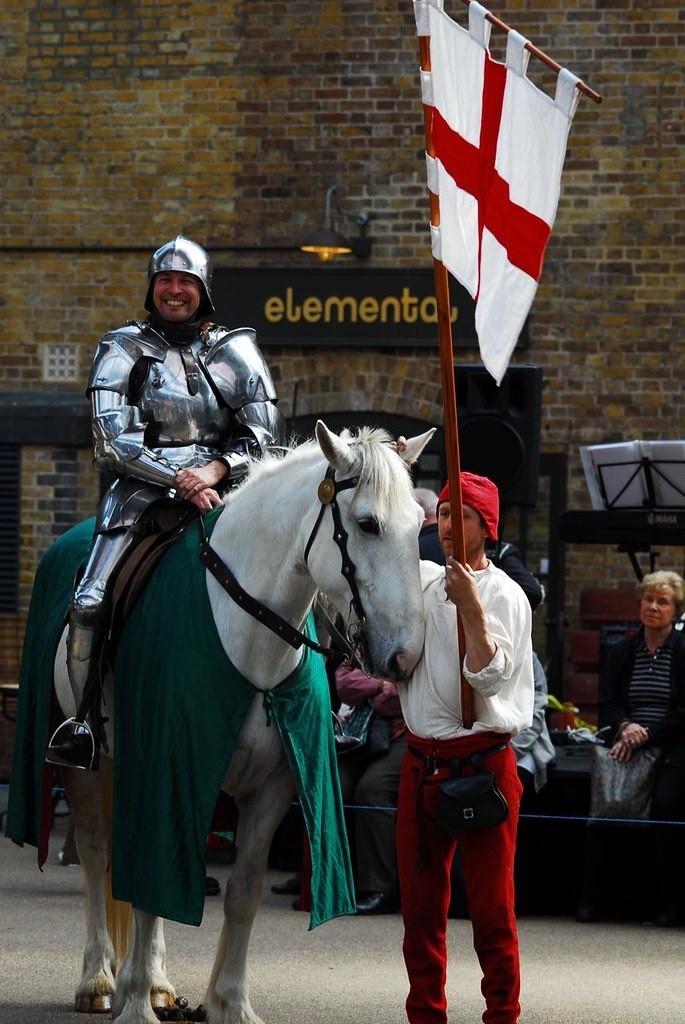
[585,745,659,828]
[407,740,510,835]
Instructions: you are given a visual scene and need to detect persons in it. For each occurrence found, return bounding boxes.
[328,471,559,1024]
[595,569,684,786]
[58,233,293,751]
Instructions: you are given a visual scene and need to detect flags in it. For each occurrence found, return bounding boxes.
[411,0,583,398]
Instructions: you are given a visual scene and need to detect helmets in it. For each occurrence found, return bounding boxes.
[140,234,215,319]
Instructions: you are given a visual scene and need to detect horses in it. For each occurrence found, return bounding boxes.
[40,418,437,1024]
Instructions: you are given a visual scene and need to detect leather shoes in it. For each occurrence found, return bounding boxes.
[267,871,301,894]
[45,719,101,774]
[354,891,401,916]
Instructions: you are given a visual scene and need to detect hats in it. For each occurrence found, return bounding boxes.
[435,470,500,542]
